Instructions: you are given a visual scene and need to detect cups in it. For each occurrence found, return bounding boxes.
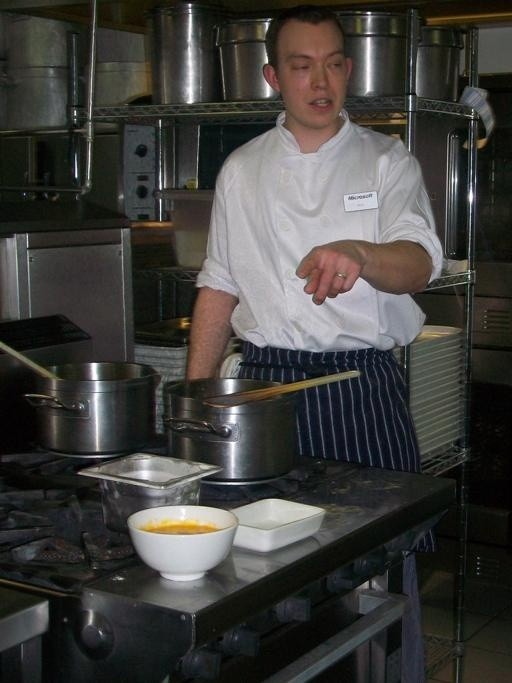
[411,20,463,103]
[156,6,218,102]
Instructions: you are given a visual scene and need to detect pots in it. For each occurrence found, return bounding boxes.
[0,14,149,131]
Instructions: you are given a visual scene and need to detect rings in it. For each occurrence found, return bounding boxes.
[333,269,346,279]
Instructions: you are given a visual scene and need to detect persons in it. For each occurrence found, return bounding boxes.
[183,0,442,493]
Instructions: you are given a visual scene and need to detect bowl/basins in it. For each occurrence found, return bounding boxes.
[230,497,325,553]
[127,505,240,582]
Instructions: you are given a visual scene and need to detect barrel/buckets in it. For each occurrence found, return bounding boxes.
[336,9,409,98]
[216,17,278,100]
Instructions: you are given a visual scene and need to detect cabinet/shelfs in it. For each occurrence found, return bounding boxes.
[123,1,481,683]
